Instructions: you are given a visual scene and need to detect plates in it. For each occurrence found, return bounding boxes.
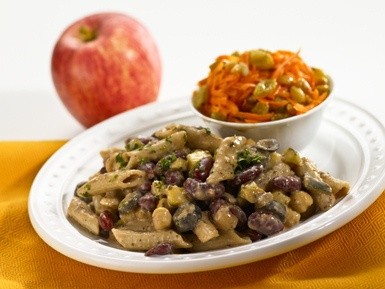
[26,93,385,276]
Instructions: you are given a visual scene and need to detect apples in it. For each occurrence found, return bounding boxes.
[50,11,162,129]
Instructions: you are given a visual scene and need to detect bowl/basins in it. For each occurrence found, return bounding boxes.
[189,70,335,154]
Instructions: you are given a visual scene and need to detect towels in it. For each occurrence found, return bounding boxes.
[1,140,385,289]
